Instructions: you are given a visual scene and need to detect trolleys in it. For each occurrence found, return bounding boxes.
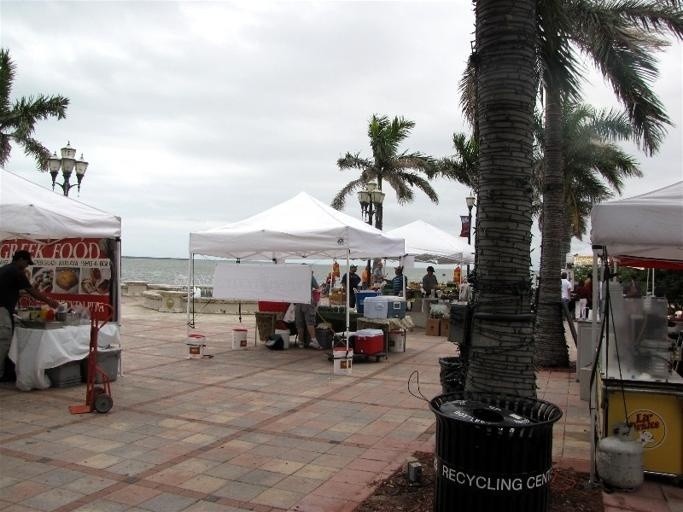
[324,323,390,363]
[67,300,115,416]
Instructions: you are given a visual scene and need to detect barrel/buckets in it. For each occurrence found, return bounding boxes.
[234,329,248,350]
[334,347,353,378]
[188,333,205,360]
[389,331,405,352]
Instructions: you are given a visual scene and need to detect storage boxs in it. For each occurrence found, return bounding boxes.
[304,325,332,351]
[440,318,449,337]
[384,294,406,319]
[353,289,381,313]
[362,297,387,321]
[425,318,438,337]
[46,359,82,389]
[81,350,121,384]
[332,332,356,350]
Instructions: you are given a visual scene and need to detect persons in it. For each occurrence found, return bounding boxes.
[558,271,604,320]
[421,266,440,304]
[0,250,58,383]
[283,263,408,351]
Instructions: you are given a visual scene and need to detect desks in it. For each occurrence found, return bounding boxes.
[7,311,118,393]
[354,327,384,356]
[251,310,285,345]
[304,308,362,334]
[356,313,414,338]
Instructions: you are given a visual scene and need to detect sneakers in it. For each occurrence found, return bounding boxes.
[298,341,303,347]
[309,338,323,349]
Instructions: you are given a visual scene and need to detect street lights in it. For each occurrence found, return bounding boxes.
[47,141,89,199]
[466,192,475,283]
[357,179,384,291]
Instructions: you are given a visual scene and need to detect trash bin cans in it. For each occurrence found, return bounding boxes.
[447,305,469,342]
[439,357,467,395]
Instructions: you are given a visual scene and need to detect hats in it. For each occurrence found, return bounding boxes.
[16,249,34,264]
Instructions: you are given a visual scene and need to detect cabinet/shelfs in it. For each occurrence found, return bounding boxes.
[576,317,604,404]
[588,348,683,490]
